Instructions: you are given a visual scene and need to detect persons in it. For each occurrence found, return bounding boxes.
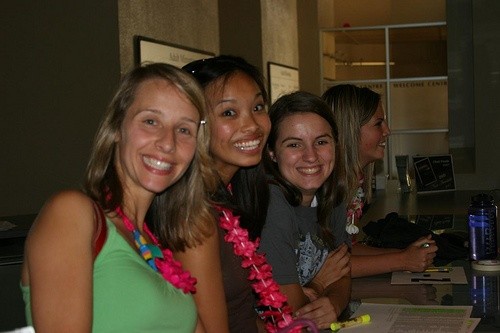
[179,54,270,333]
[255,90,352,333]
[322,83,438,278]
[19,63,229,333]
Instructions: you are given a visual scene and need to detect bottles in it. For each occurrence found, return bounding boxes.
[467,193,497,263]
[470,259,500,324]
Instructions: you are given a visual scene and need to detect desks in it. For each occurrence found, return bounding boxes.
[350,190,500,333]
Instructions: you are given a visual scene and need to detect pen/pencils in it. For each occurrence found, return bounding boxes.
[408,268,448,274]
[411,277,450,284]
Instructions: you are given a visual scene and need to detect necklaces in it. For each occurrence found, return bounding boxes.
[105,186,199,294]
[345,177,367,234]
[213,184,302,333]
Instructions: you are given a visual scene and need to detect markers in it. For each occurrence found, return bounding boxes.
[330,314,370,331]
